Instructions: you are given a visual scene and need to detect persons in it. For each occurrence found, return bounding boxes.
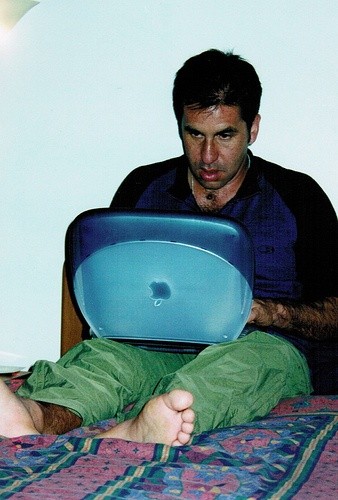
[0,47,338,446]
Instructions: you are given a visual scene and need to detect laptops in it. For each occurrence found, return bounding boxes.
[65,207,259,353]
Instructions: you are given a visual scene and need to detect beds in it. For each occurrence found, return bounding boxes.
[0,261,338,500]
